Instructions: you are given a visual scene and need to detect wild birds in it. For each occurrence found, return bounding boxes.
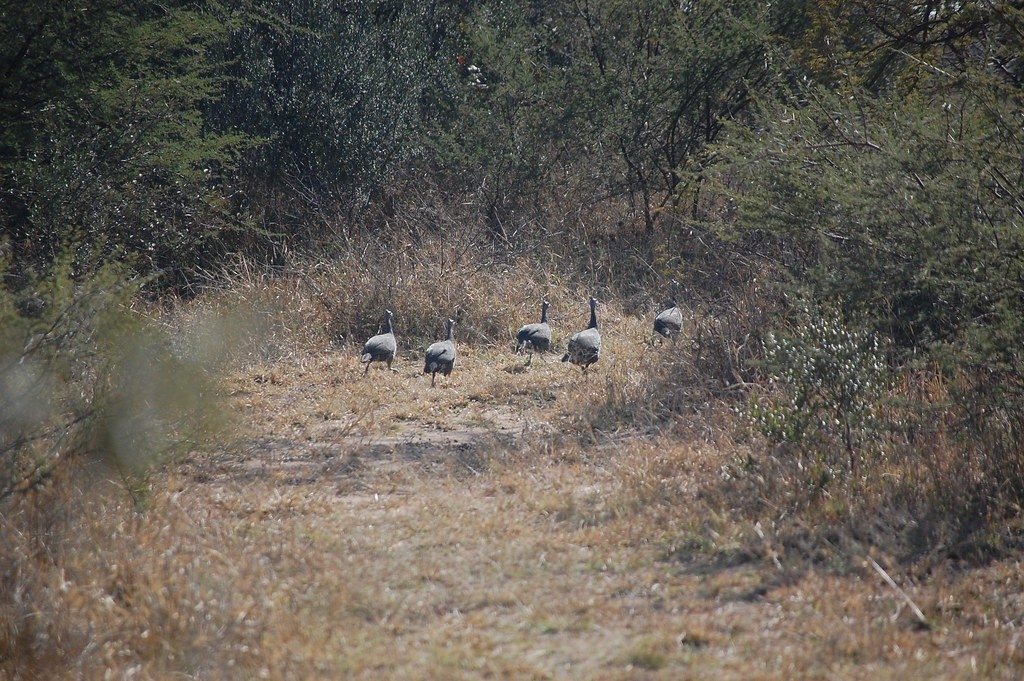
[561,295,602,373]
[360,308,398,374]
[423,318,457,388]
[653,279,685,347]
[513,300,554,367]
